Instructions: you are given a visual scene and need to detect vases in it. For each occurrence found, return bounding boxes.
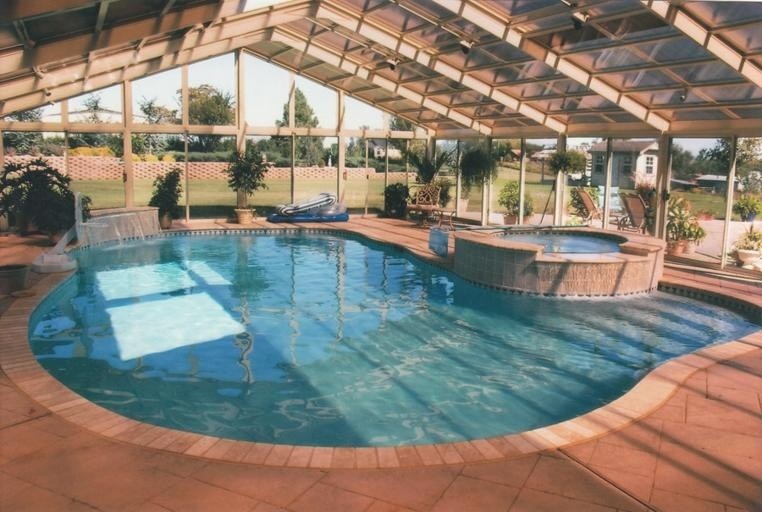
[0,263,28,292]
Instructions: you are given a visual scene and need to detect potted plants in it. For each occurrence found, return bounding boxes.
[219,139,272,225]
[498,181,534,225]
[147,168,184,228]
[666,199,719,255]
[0,156,92,244]
[452,139,512,212]
[732,192,762,222]
[737,228,762,270]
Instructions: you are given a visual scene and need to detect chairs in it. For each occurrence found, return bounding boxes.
[577,189,618,226]
[406,185,442,228]
[618,193,649,234]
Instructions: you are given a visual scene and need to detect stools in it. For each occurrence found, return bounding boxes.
[432,208,457,231]
